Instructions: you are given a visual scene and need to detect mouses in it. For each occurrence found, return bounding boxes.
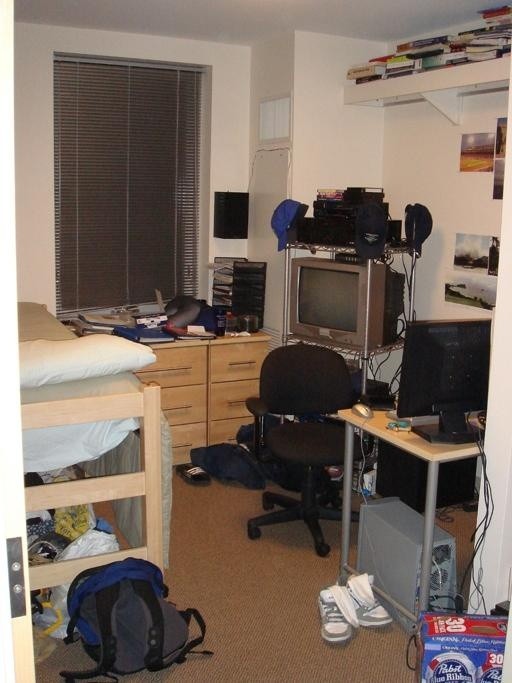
[351,403,374,418]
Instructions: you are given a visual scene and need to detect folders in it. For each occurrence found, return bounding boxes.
[115,327,174,343]
[71,319,114,336]
[79,312,125,326]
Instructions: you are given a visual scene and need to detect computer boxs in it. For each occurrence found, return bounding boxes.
[358,494,455,635]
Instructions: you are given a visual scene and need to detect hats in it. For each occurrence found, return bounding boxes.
[271,200,309,252]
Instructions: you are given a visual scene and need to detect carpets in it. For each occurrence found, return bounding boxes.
[24,464,478,679]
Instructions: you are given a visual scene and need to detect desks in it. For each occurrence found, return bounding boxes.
[334,406,487,641]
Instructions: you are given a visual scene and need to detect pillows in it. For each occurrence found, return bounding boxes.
[18,331,157,389]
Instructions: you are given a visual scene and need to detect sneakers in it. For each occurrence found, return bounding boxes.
[338,574,394,630]
[318,588,352,645]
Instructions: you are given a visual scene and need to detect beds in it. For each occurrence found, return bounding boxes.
[16,300,163,594]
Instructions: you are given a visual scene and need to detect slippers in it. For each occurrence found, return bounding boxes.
[177,464,211,486]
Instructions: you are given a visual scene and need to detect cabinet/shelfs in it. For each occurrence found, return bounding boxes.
[135,337,208,469]
[206,332,273,447]
[344,53,512,125]
[281,243,420,504]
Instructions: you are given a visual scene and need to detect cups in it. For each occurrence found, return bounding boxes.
[215,317,227,336]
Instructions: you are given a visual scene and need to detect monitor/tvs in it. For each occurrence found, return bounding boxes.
[396,319,491,445]
[289,256,405,347]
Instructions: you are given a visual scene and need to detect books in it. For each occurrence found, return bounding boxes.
[347,9,512,83]
[211,264,233,306]
[318,189,346,201]
[73,314,215,345]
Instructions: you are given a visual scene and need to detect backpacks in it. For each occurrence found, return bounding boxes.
[56,556,212,677]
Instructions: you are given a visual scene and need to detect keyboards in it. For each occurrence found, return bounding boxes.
[387,408,483,425]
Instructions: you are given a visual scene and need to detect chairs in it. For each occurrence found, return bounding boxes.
[244,342,364,556]
